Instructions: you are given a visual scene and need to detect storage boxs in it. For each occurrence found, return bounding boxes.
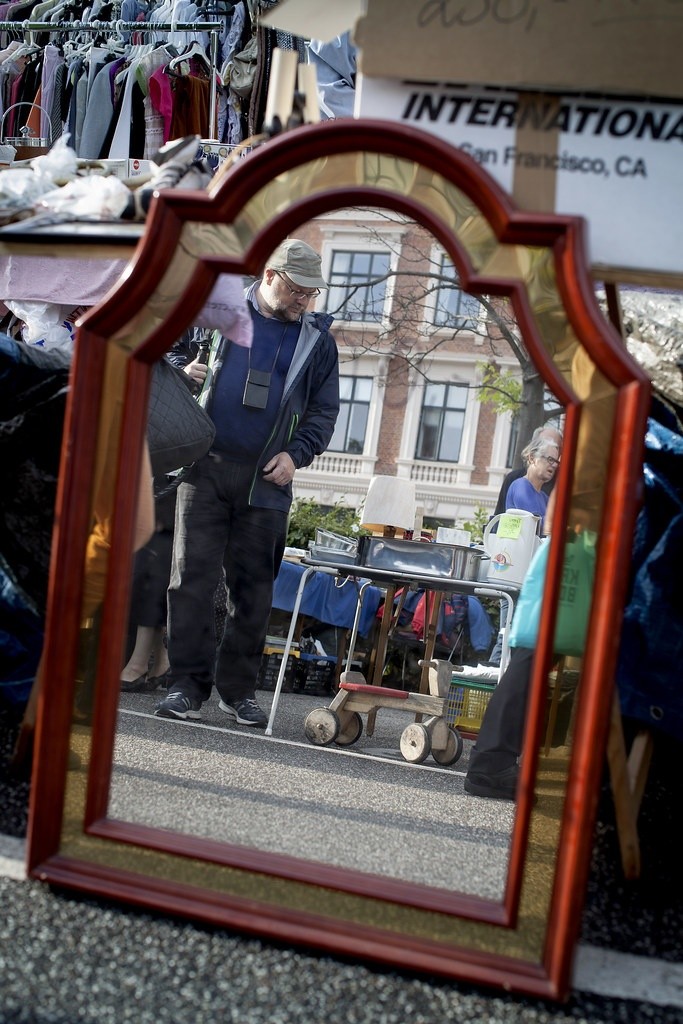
[99,159,151,178]
[254,0,682,276]
[200,142,251,158]
[258,648,363,696]
[444,678,496,734]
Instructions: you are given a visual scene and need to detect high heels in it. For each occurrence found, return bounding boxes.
[144,666,174,690]
[120,670,148,692]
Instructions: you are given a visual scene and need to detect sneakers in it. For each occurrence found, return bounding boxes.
[154,691,203,720]
[218,697,268,725]
[464,760,539,805]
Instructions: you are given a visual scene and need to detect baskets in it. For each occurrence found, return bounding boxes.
[444,679,495,734]
[0,102,53,160]
[258,635,338,696]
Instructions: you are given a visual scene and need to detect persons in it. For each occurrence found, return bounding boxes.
[118,240,339,727]
[464,428,563,803]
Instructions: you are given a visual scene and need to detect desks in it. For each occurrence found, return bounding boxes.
[271,562,381,693]
[264,556,519,737]
[0,219,146,305]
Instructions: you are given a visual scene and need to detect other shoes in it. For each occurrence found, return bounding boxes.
[73,706,90,720]
[66,750,82,770]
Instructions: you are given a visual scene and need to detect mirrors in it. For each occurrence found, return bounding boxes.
[25,120,654,1005]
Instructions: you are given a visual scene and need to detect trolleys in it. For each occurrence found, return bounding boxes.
[263,555,522,768]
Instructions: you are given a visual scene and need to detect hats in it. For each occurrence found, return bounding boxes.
[266,238,330,291]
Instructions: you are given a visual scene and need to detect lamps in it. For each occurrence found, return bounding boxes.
[360,474,416,538]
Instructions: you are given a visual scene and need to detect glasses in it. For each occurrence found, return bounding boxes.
[542,456,560,465]
[274,270,321,300]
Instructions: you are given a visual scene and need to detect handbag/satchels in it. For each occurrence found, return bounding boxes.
[507,529,598,657]
[243,369,272,409]
[229,38,258,98]
[145,355,216,477]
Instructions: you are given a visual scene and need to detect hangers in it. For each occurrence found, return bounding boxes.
[4,18,224,97]
[191,0,235,16]
[199,146,221,171]
